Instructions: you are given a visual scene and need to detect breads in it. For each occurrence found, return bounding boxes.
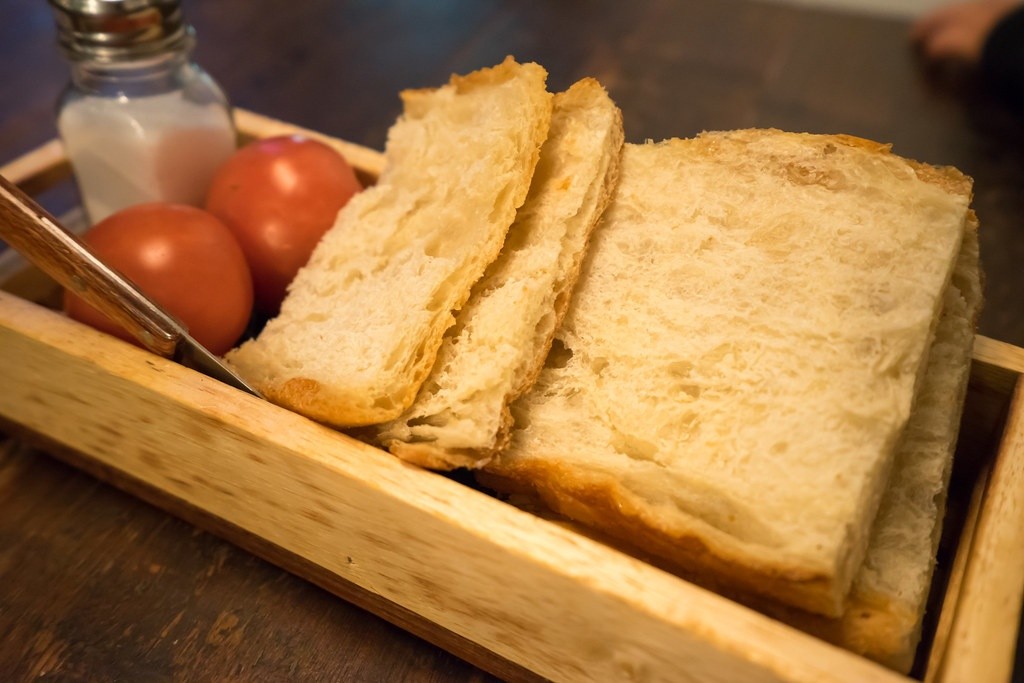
[226,56,988,674]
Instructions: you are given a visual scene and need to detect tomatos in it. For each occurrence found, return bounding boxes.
[205,130,365,319]
[63,198,251,359]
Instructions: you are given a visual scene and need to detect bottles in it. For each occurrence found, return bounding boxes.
[54,0,240,226]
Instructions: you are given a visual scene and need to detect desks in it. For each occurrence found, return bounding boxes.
[0,0,1024,683]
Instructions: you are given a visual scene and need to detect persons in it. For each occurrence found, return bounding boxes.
[907,0,1024,162]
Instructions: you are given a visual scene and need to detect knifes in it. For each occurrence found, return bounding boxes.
[1,176,263,398]
